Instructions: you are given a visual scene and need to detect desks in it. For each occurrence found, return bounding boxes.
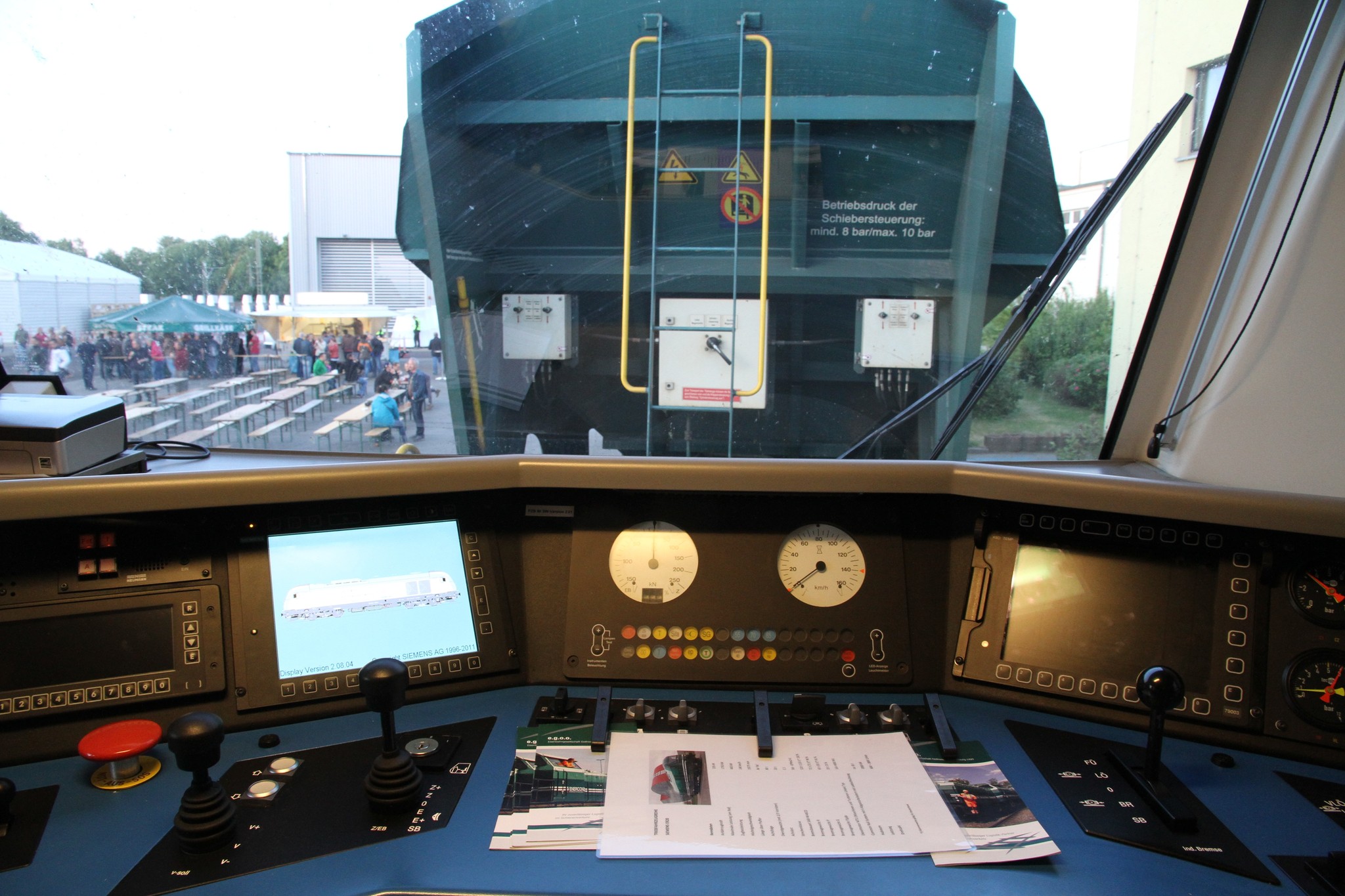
[86,367,410,442]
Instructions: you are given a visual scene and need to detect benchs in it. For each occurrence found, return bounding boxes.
[123,402,155,420]
[188,399,230,427]
[278,377,300,388]
[235,386,272,405]
[244,378,267,388]
[131,420,180,441]
[257,399,280,412]
[202,419,236,441]
[248,417,296,449]
[366,417,406,452]
[399,400,412,419]
[291,399,323,432]
[319,385,354,410]
[314,419,344,451]
[126,388,159,401]
[158,387,204,413]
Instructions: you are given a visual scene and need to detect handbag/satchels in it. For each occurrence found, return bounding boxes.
[289,353,298,373]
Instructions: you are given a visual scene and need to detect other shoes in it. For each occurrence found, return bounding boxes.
[410,434,425,442]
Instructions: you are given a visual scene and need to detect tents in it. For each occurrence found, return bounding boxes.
[89,294,256,332]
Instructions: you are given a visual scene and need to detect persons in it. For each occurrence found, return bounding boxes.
[346,317,364,339]
[0,331,4,353]
[371,384,408,447]
[413,316,421,348]
[14,323,261,391]
[293,327,443,412]
[405,357,428,443]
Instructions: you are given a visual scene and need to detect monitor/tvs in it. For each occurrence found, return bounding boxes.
[267,516,478,681]
[999,535,1218,697]
